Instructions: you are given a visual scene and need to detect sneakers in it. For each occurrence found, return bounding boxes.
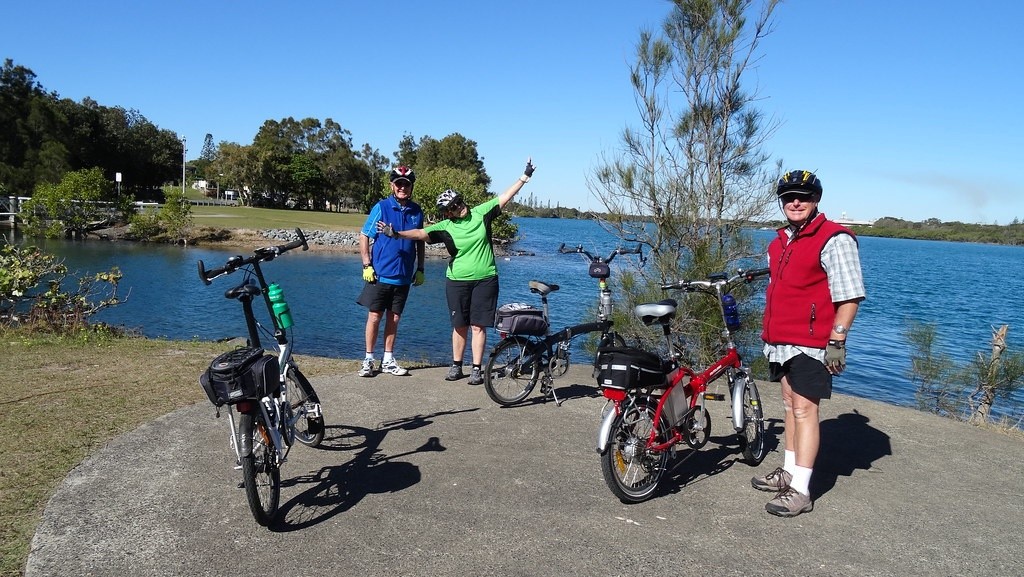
[445,365,464,381]
[359,358,375,377]
[468,368,483,385]
[378,355,408,376]
[750,467,794,492]
[766,484,814,517]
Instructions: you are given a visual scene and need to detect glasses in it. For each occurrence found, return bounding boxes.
[394,181,412,188]
[782,193,815,202]
[449,197,463,212]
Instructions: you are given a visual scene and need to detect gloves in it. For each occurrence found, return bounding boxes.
[412,267,424,286]
[362,265,378,282]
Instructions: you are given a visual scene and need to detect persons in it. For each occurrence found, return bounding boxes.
[356,166,426,377]
[374,154,536,384]
[751,170,866,517]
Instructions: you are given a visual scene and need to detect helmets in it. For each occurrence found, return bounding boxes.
[777,170,823,203]
[435,189,460,211]
[390,166,415,185]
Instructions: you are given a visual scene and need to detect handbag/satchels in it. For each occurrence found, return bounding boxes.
[494,303,548,337]
[597,345,668,390]
[197,346,282,408]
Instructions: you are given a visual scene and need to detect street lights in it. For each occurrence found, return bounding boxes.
[181,135,186,209]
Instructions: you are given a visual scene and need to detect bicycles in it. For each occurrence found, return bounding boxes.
[596,268,770,505]
[479,241,648,406]
[198,226,326,527]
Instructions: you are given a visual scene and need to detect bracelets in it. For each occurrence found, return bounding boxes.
[362,262,372,269]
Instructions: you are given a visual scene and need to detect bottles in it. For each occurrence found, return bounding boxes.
[722,294,739,331]
[602,286,612,315]
[267,281,293,329]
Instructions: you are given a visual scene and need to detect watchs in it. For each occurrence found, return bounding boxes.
[832,325,848,335]
